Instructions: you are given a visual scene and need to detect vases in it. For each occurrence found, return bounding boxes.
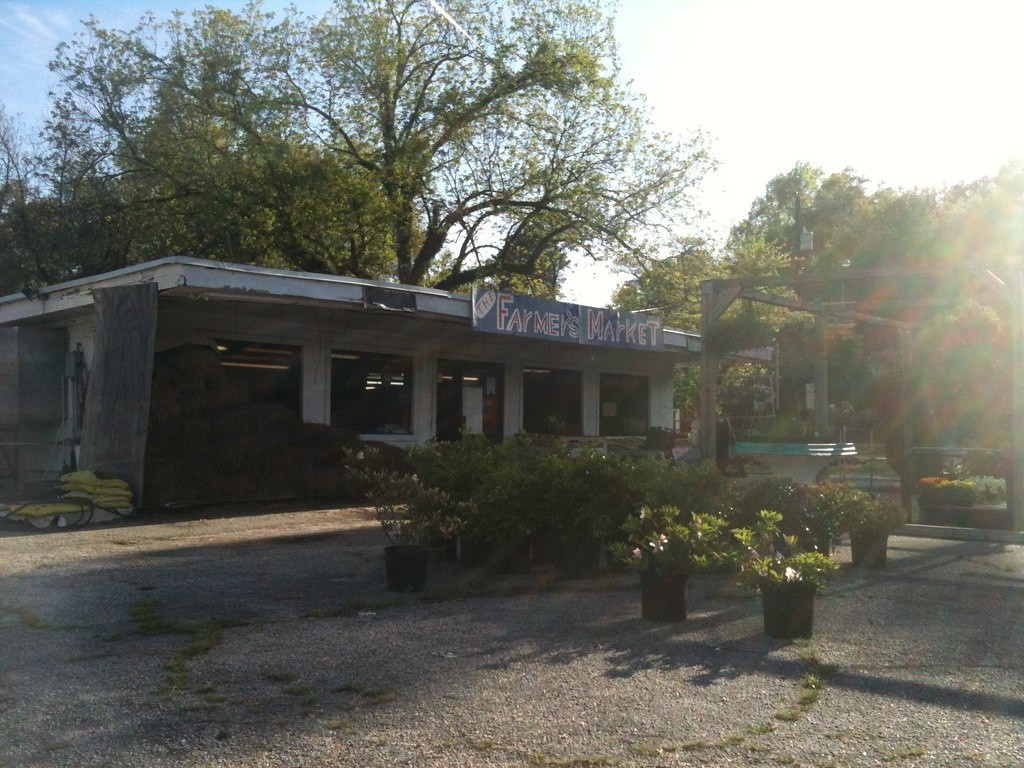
[850,532,889,567]
[803,536,830,558]
[973,506,1005,529]
[381,546,427,593]
[421,526,456,561]
[757,585,815,638]
[917,497,942,524]
[459,531,489,554]
[939,506,971,525]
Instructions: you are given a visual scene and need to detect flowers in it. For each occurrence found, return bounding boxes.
[732,511,841,593]
[917,476,978,505]
[637,569,686,623]
[844,485,907,534]
[349,428,494,549]
[608,506,731,571]
